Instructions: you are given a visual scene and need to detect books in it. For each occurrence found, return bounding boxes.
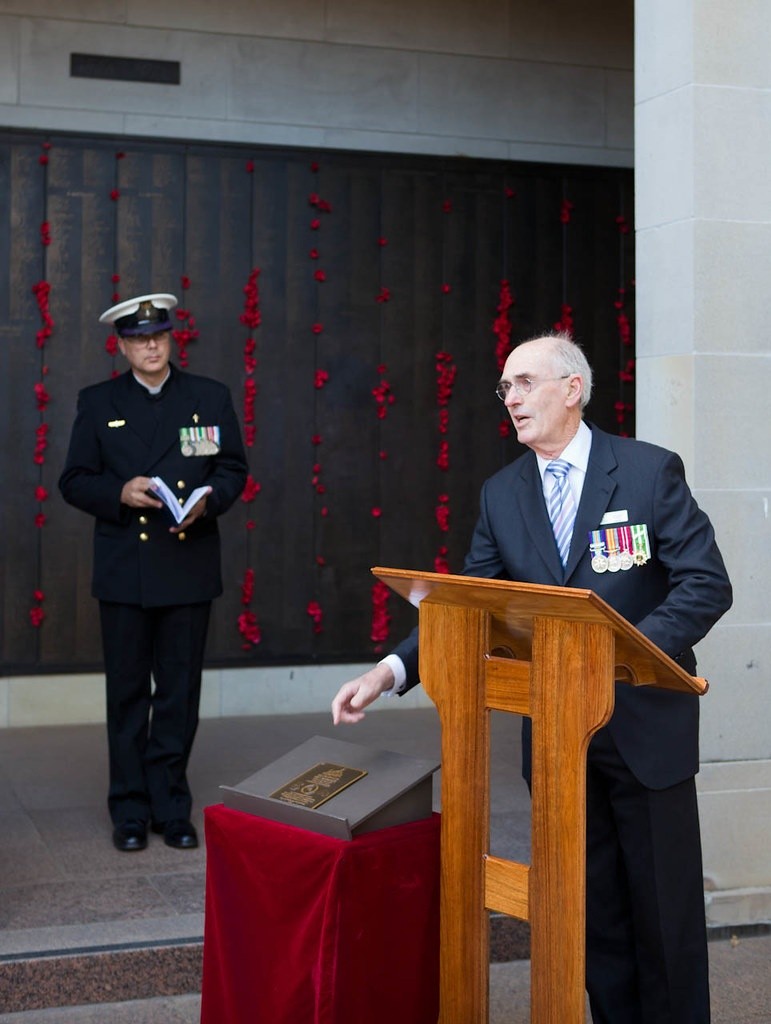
[145,477,209,529]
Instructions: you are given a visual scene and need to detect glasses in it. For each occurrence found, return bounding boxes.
[123,332,170,348]
[494,374,571,401]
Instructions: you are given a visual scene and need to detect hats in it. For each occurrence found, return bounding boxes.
[99,293,178,336]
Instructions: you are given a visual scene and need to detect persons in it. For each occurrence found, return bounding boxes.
[58,291,249,851]
[331,330,733,1024]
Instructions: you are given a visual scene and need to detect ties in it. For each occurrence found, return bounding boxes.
[546,461,577,570]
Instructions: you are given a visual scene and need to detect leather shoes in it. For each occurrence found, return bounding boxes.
[149,815,199,848]
[113,816,150,849]
